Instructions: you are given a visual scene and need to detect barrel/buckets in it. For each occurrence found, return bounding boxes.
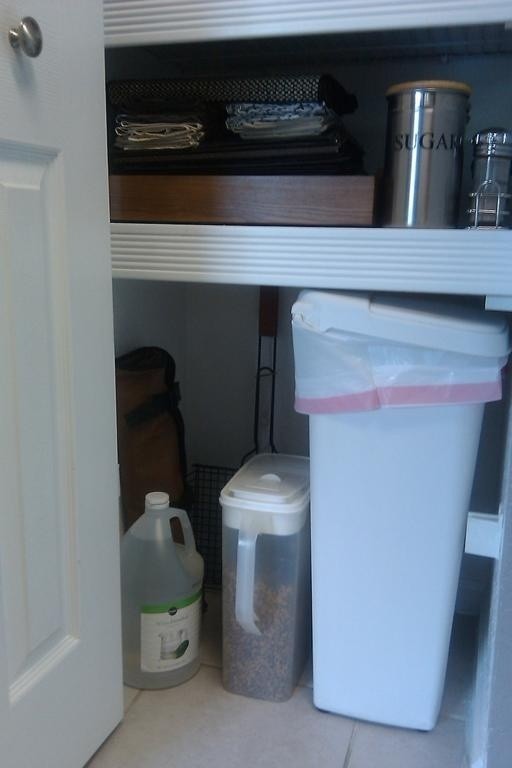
[120,493,205,691]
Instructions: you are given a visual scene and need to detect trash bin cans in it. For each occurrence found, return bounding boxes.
[292,290,509,730]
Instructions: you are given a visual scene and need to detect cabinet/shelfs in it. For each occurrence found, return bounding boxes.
[99,0,512,311]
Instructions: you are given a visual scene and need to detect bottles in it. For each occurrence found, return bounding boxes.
[469,128,511,227]
[382,80,471,229]
